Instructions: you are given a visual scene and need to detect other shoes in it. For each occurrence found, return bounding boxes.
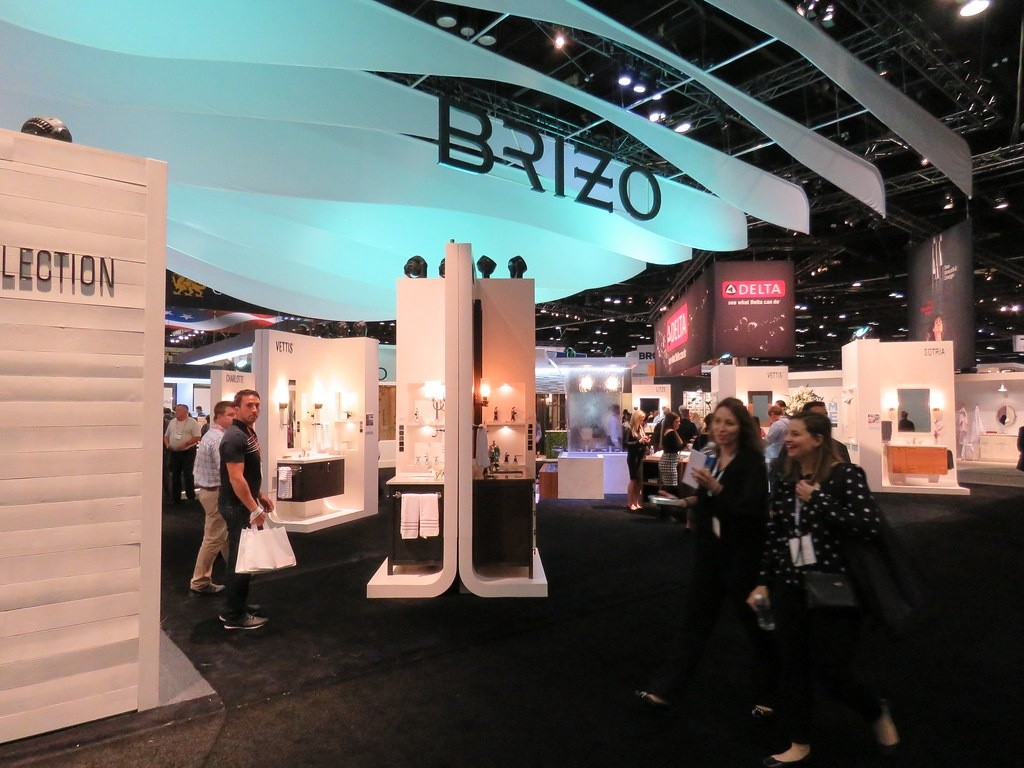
[627,505,646,513]
[870,698,899,756]
[751,704,775,727]
[634,690,679,710]
[762,748,814,768]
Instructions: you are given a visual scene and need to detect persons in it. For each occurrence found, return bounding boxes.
[504,452,509,462]
[623,405,697,495]
[511,407,516,421]
[625,411,650,512]
[899,411,915,431]
[1015,426,1024,473]
[630,398,899,768]
[999,416,1006,425]
[537,423,544,457]
[218,389,275,630]
[513,456,517,463]
[491,441,498,454]
[494,407,497,421]
[190,401,235,592]
[608,405,622,448]
[163,404,210,509]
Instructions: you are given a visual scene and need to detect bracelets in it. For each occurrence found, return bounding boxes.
[186,443,188,445]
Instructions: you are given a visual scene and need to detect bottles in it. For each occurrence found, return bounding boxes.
[755,594,775,630]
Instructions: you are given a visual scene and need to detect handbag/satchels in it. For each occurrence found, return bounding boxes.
[235,508,296,574]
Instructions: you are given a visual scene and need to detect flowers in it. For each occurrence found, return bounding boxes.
[782,383,822,419]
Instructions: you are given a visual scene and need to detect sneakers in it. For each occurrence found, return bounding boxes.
[224,612,269,630]
[189,583,226,597]
[219,614,226,621]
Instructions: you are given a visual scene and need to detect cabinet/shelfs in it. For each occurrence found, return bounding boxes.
[387,485,444,575]
[887,446,948,475]
[470,478,538,579]
[276,458,347,502]
[980,434,1020,463]
